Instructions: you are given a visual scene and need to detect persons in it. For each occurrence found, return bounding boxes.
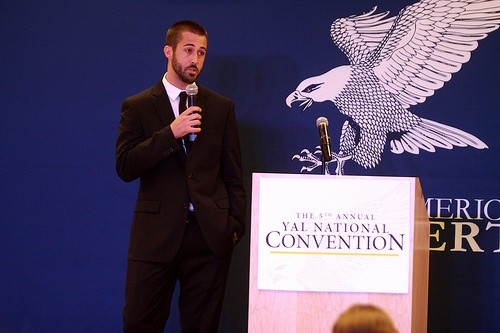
[330,304,401,332]
[115,19,246,332]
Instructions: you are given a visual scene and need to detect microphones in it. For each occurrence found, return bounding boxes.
[186,84,198,141]
[317,117,333,162]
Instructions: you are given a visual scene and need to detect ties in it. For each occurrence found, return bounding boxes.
[179,92,191,154]
